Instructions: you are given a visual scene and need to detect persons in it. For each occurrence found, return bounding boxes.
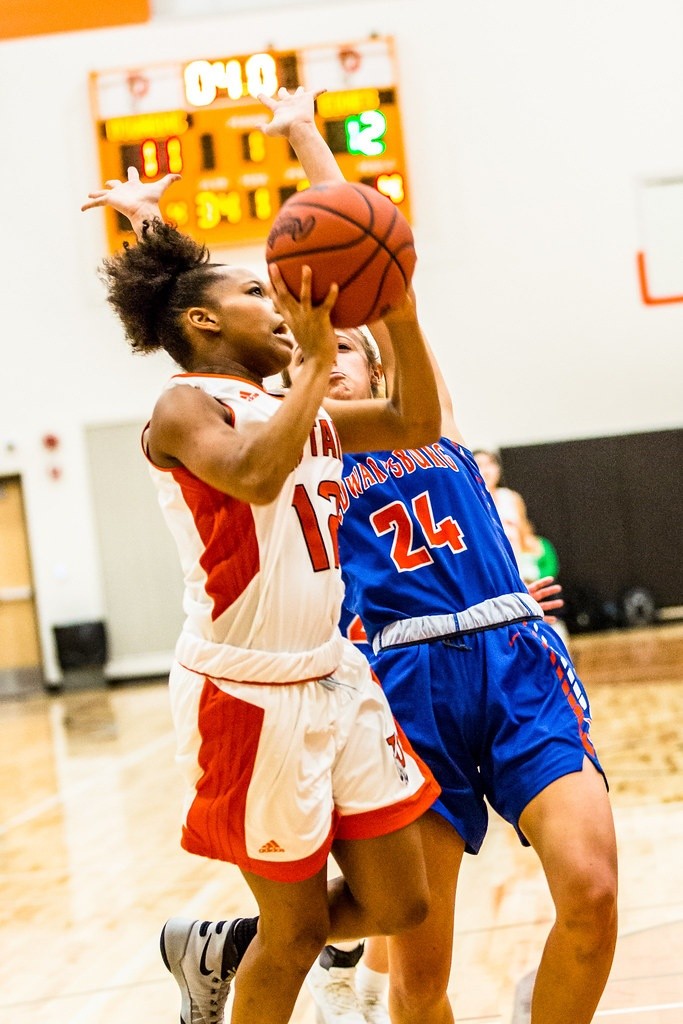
[472,448,544,581]
[310,574,565,1024]
[81,84,617,1024]
[96,213,441,1023]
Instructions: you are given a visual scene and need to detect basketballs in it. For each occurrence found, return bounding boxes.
[265,180,418,330]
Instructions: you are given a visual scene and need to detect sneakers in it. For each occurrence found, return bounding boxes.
[158,913,240,1024]
[316,942,394,1024]
